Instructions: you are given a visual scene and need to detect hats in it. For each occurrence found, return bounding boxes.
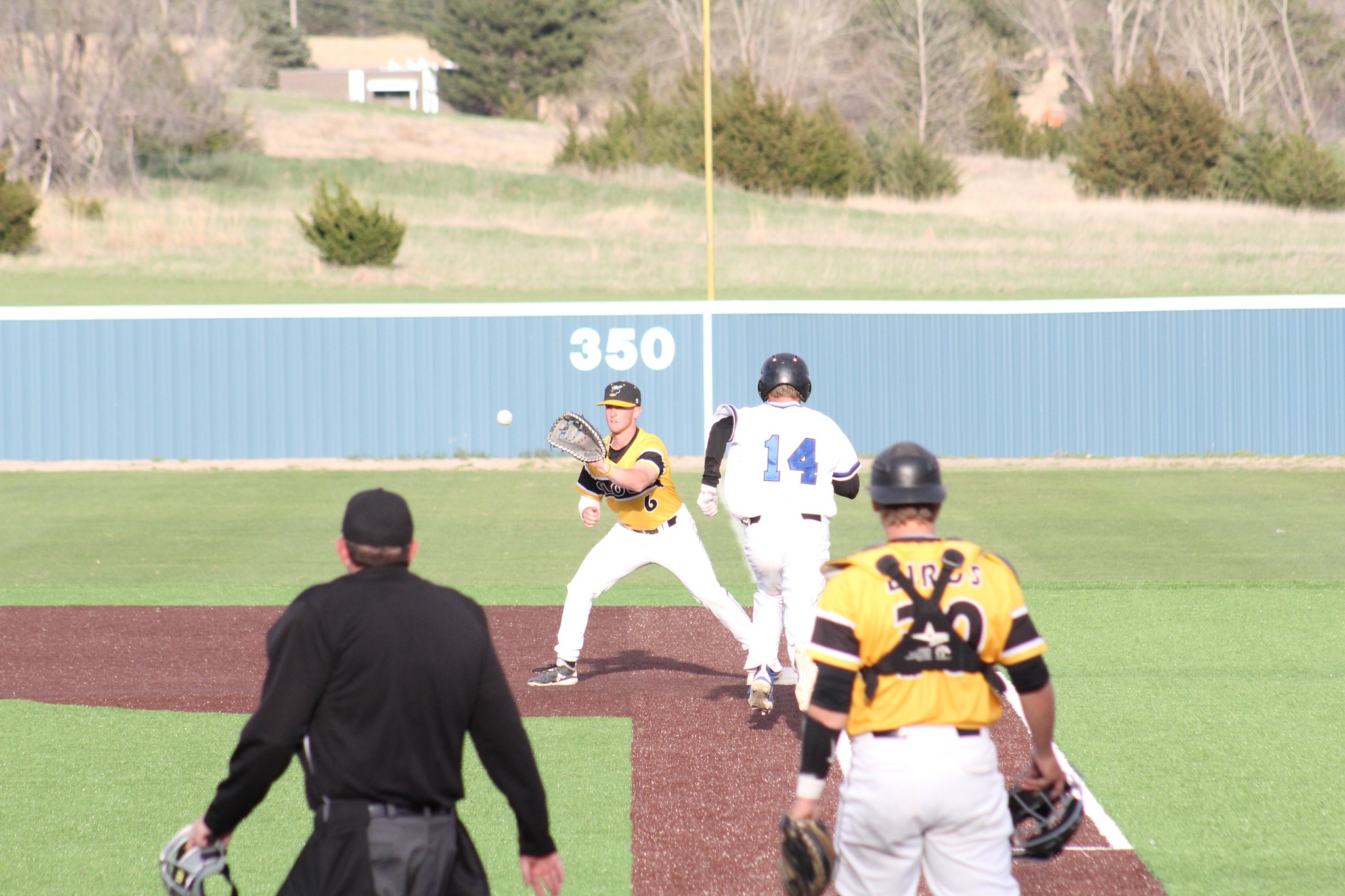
[596,380,641,408]
[343,490,412,546]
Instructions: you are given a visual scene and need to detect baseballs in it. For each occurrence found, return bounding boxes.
[497,409,513,425]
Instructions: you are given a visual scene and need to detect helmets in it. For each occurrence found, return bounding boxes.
[870,442,947,504]
[758,352,811,403]
[1008,774,1085,859]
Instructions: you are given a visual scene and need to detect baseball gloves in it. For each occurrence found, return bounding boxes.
[547,412,607,463]
[778,811,836,896]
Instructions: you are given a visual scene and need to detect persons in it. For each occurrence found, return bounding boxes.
[526,379,783,687]
[183,488,569,896]
[786,441,1068,896]
[696,352,862,717]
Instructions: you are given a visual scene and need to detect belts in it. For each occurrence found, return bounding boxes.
[748,513,822,525]
[631,516,677,534]
[323,796,453,824]
[872,728,981,738]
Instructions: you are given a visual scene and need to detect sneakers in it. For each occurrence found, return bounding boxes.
[774,669,782,678]
[747,664,775,715]
[528,658,578,686]
[794,642,818,712]
